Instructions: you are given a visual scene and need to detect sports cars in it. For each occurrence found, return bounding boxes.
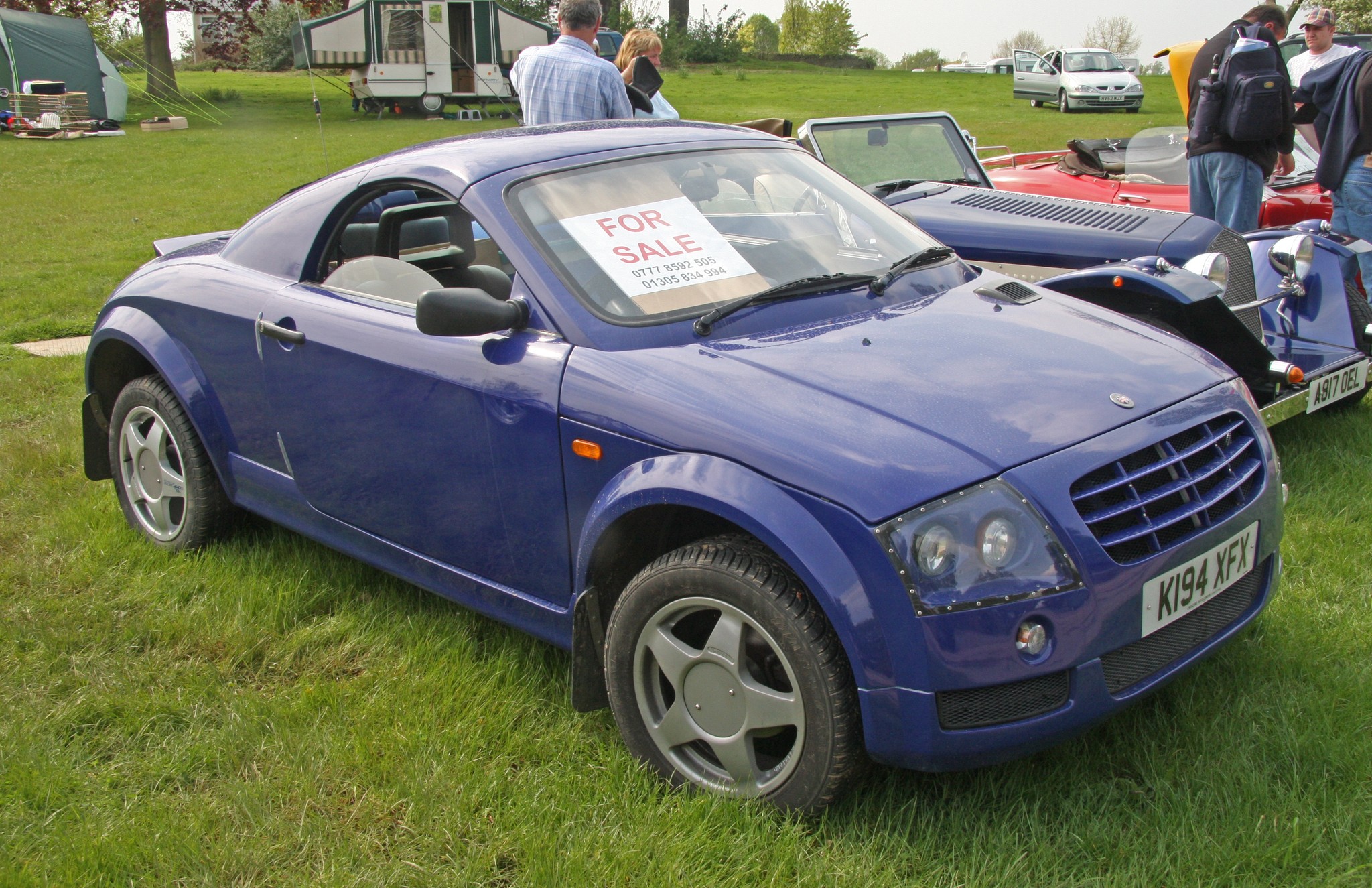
[80,117,1288,830]
[733,115,1372,430]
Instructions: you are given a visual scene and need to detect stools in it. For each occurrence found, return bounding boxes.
[456,110,483,121]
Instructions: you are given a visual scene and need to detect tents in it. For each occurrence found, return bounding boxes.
[0,7,128,129]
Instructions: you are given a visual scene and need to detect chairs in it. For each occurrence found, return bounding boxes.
[375,201,514,300]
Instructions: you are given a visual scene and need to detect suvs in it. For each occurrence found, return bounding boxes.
[551,26,626,73]
[1275,32,1372,66]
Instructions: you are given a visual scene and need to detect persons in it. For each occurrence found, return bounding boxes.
[1285,9,1362,87]
[509,0,680,126]
[352,98,360,112]
[1186,3,1296,233]
[1291,48,1372,306]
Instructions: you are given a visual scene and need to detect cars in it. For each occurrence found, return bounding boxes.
[1012,47,1144,113]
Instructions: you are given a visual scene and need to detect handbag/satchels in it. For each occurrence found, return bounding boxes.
[1057,134,1188,178]
[98,120,121,130]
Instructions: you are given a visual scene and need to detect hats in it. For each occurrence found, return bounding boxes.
[1299,8,1336,30]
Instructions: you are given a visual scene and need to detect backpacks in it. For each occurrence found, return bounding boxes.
[1190,24,1286,142]
